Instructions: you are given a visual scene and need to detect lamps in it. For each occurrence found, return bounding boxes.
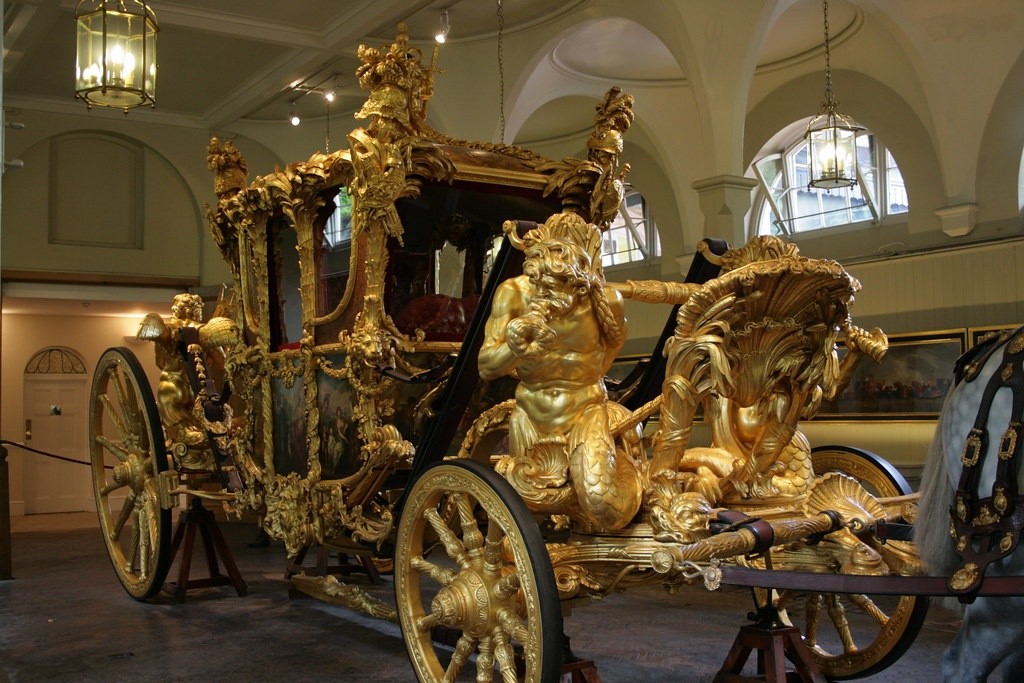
[124,335,150,344]
[803,0,859,194]
[73,0,159,117]
[435,7,451,44]
[324,76,341,102]
[288,101,300,126]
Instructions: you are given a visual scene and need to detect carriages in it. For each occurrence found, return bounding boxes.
[87,23,1024,683]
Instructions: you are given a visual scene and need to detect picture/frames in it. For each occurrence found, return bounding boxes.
[969,324,1024,381]
[604,353,704,423]
[798,328,968,423]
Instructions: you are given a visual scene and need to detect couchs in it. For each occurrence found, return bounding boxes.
[396,292,482,343]
